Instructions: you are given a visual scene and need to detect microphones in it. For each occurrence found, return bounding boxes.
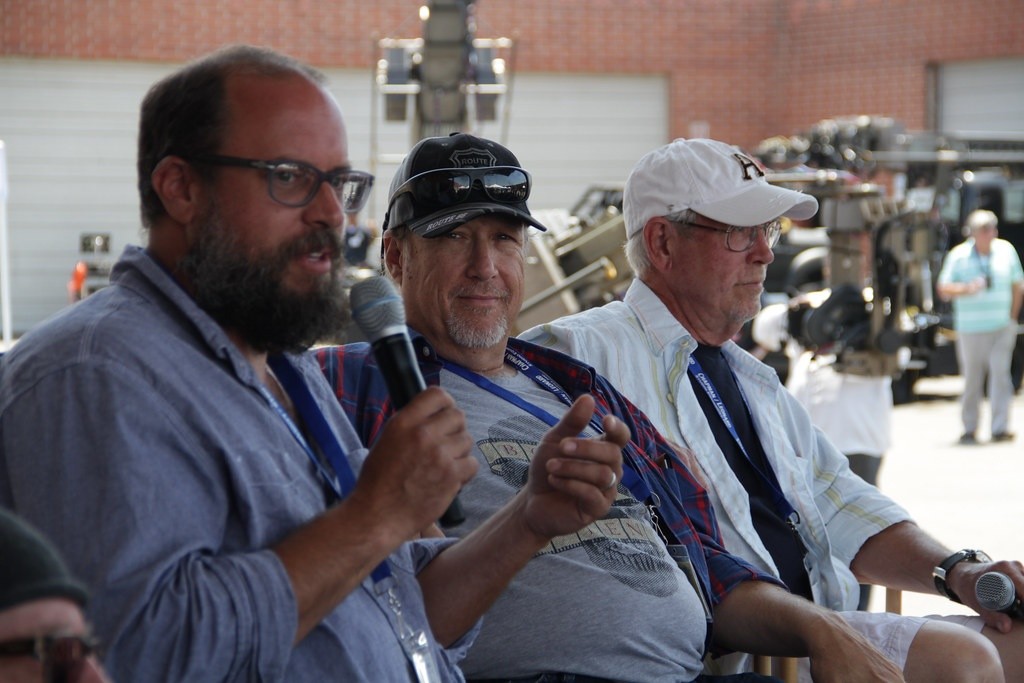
[350,276,467,529]
[974,571,1024,617]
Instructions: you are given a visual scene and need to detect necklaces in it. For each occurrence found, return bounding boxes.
[972,245,994,289]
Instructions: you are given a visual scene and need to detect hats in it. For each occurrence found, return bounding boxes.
[622,138,817,239]
[0,514,93,612]
[382,129,546,238]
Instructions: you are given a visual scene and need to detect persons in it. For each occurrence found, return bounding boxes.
[0,503,107,683]
[307,133,904,683]
[514,138,1024,683]
[0,42,631,683]
[937,209,1024,444]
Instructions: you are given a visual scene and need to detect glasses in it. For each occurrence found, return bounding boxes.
[675,217,779,249]
[0,627,106,683]
[207,151,376,214]
[388,166,534,210]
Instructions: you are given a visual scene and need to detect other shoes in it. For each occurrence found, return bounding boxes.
[959,433,979,444]
[991,431,1015,442]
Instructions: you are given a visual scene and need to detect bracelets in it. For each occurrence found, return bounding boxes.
[931,549,993,605]
